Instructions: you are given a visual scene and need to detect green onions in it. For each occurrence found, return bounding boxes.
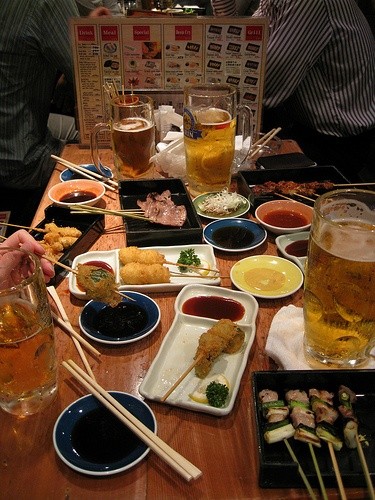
[259,397,360,450]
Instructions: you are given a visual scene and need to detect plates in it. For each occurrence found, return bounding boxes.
[231,255,304,299]
[78,291,161,346]
[118,179,203,248]
[276,232,310,273]
[48,164,114,209]
[53,390,157,476]
[140,284,258,416]
[253,369,375,490]
[29,205,221,301]
[54,391,156,477]
[241,152,353,205]
[255,200,314,235]
[203,219,267,251]
[59,164,113,183]
[193,190,250,220]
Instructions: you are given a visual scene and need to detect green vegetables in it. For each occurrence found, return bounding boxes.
[204,381,229,408]
[177,248,201,272]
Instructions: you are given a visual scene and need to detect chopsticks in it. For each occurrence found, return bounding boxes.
[50,154,118,194]
[37,285,203,483]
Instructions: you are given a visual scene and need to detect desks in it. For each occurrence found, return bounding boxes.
[0,138,375,500]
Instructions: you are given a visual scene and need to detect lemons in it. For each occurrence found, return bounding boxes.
[193,260,211,276]
[188,373,230,403]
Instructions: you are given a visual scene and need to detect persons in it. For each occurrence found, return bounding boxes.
[210,0,375,138]
[0,0,125,190]
[0,228,56,292]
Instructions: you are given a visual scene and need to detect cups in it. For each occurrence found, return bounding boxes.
[89,95,155,177]
[0,247,58,417]
[303,190,375,370]
[184,83,255,197]
